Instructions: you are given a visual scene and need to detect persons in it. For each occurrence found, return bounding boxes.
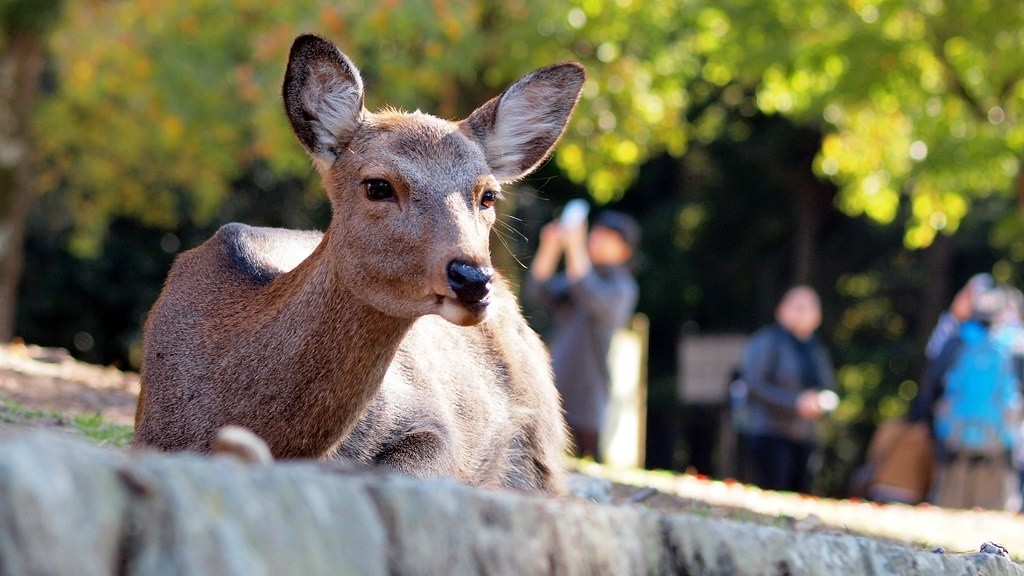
[869,415,933,503]
[521,211,641,465]
[925,274,1024,515]
[730,285,838,495]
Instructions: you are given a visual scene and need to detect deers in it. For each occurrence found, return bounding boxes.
[133,32,587,499]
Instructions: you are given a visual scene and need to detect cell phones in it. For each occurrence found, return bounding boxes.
[559,199,589,229]
